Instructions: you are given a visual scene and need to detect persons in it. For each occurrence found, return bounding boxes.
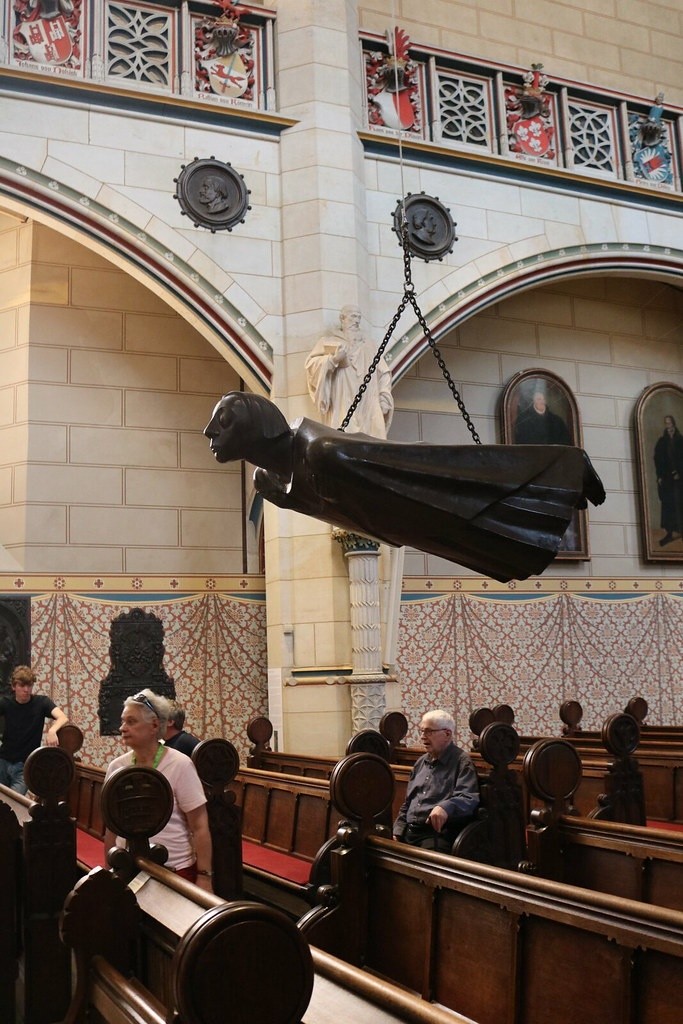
[98,688,214,896]
[0,665,70,797]
[159,699,203,758]
[511,390,580,551]
[653,414,683,546]
[201,391,607,585]
[390,709,484,848]
[303,304,395,532]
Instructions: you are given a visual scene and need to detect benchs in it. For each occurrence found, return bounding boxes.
[0,723,682,1024]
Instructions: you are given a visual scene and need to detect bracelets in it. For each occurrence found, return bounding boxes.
[194,867,214,876]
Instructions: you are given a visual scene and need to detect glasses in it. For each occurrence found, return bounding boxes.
[417,727,448,734]
[131,693,160,720]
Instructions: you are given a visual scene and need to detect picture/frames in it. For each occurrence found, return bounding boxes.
[500,367,592,563]
[633,382,682,563]
